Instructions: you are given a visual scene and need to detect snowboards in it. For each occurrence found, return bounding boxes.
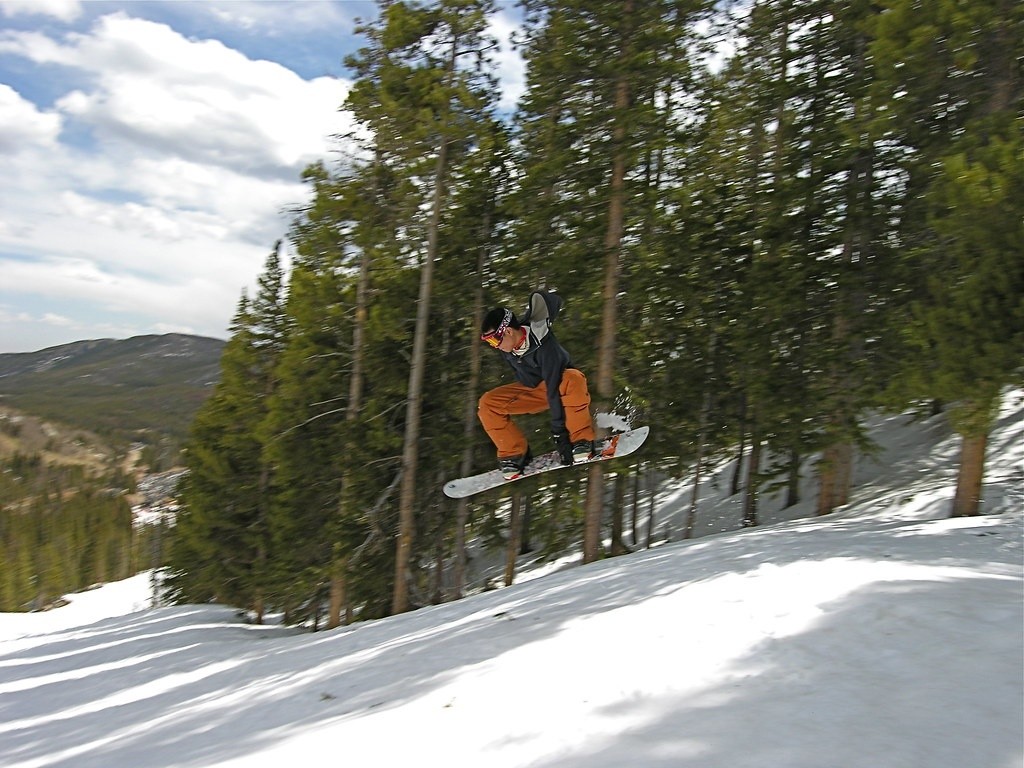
[443,426,650,499]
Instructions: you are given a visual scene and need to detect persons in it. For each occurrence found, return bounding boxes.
[477,289,595,480]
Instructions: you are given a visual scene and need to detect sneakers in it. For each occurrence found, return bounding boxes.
[572,439,594,461]
[499,445,533,479]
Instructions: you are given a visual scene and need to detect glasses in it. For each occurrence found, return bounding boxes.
[481,333,503,348]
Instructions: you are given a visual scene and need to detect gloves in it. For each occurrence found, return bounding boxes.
[551,428,573,465]
